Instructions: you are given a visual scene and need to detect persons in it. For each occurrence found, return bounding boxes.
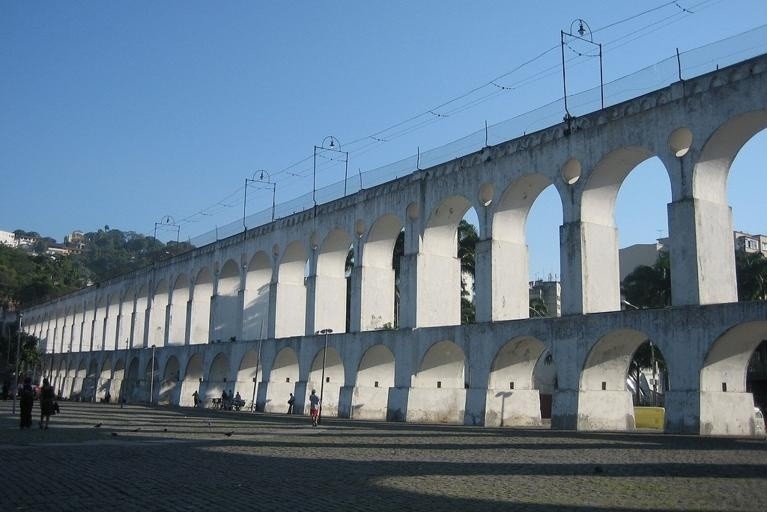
[287,393,295,414]
[192,391,199,407]
[309,389,320,426]
[220,390,242,412]
[3,377,56,431]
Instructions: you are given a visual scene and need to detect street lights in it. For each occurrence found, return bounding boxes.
[318,328,333,422]
[11,313,24,415]
[150,344,156,403]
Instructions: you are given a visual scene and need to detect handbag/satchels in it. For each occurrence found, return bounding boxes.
[199,400,201,403]
[50,402,59,414]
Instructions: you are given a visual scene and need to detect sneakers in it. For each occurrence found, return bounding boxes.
[312,421,317,426]
